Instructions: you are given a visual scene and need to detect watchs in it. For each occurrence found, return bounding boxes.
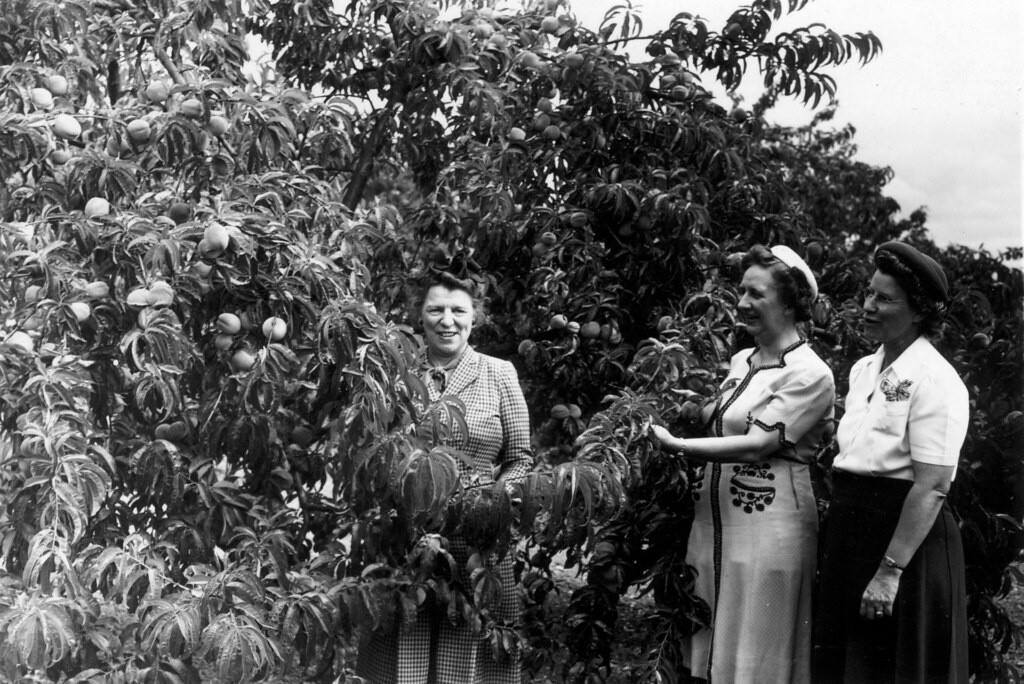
[882,555,905,570]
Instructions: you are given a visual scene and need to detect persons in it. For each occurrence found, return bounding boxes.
[353,271,535,684]
[648,243,836,684]
[830,242,969,684]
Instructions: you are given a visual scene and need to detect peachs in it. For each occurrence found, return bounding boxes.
[0,11,1023,479]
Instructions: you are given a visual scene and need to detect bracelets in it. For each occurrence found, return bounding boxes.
[678,437,686,456]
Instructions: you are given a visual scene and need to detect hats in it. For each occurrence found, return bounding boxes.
[874,242,949,302]
[770,244,818,304]
